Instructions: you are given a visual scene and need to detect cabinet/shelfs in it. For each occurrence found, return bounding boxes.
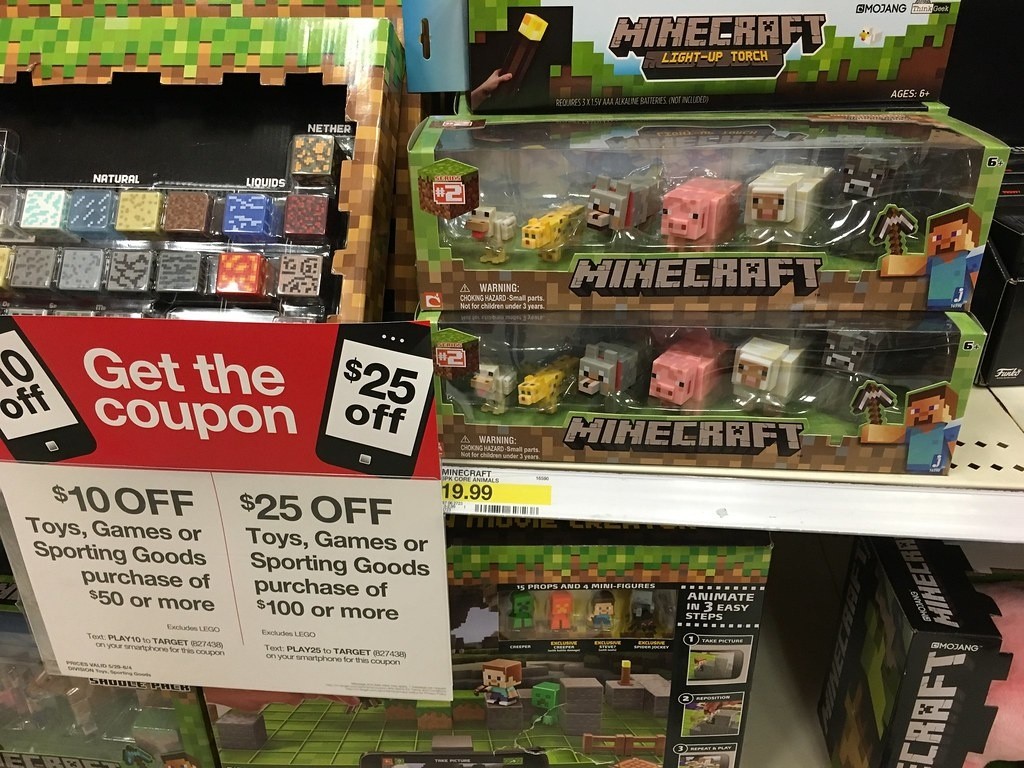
[440,385,1024,768]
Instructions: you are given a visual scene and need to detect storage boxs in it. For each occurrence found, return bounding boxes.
[412,303,988,475]
[403,0,966,109]
[816,535,1013,768]
[204,514,776,768]
[409,112,1014,315]
[967,207,1024,387]
[0,573,220,768]
[0,16,403,324]
